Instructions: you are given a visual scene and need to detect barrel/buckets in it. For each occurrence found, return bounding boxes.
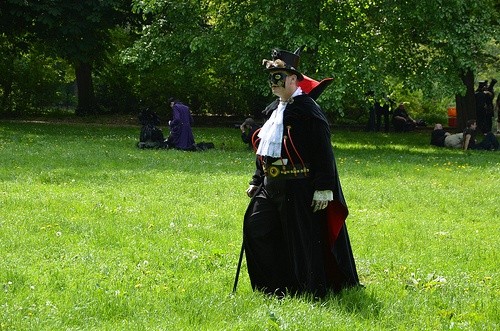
[448,108,457,127]
[457,95,492,132]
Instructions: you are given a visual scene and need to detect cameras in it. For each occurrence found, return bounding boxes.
[235,123,246,129]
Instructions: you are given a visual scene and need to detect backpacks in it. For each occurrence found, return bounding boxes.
[138,122,165,148]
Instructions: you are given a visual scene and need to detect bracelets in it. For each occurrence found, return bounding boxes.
[241,128,244,131]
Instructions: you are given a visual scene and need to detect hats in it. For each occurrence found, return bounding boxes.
[262,50,304,81]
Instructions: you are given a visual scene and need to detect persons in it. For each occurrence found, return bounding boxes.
[242,50,363,302]
[390,92,500,152]
[136,99,261,150]
